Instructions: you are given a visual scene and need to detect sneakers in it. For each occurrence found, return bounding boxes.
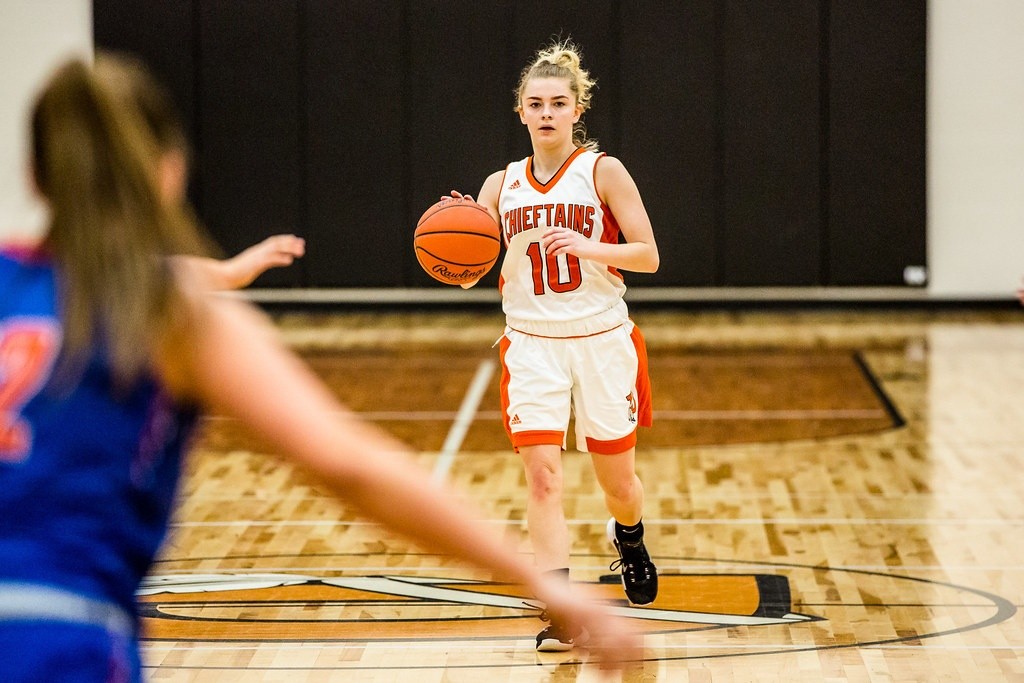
[607,514,658,605]
[522,600,589,650]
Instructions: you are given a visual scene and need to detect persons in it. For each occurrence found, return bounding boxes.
[0,50,649,683]
[442,42,659,651]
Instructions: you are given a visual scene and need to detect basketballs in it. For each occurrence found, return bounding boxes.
[414,198,501,285]
